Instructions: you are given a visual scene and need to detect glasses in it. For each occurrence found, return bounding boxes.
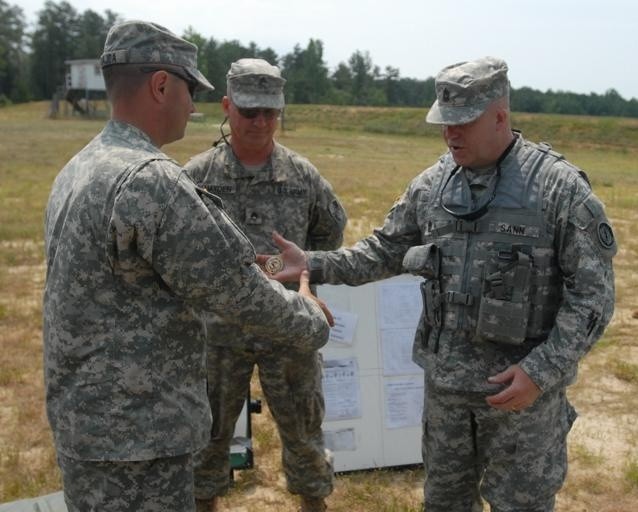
[439,174,497,221]
[236,107,279,120]
[142,67,196,97]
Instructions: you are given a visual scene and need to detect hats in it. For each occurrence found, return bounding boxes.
[102,20,215,90]
[426,58,507,125]
[225,58,286,111]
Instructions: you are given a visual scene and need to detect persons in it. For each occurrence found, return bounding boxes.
[253,56,618,512]
[43,20,334,511]
[194,57,348,511]
